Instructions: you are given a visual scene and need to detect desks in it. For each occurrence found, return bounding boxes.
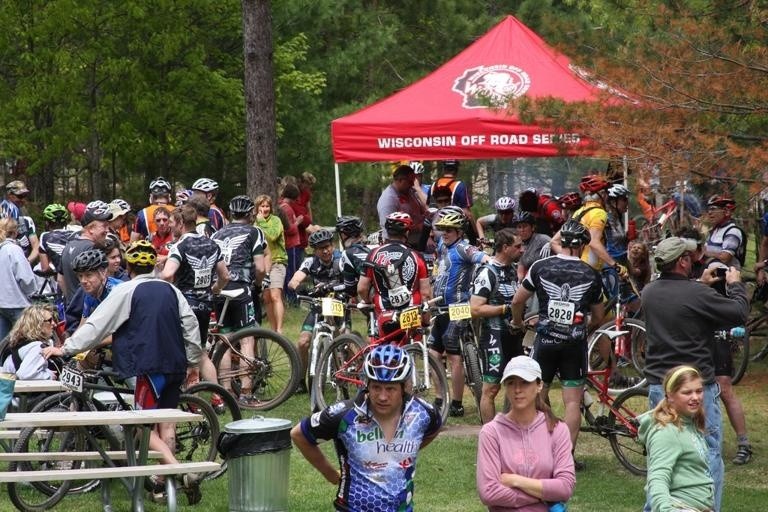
[0,407,203,511]
[14,378,67,412]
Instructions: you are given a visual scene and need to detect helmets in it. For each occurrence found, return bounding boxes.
[407,160,459,175]
[431,206,469,229]
[707,191,737,212]
[228,194,255,213]
[42,200,131,227]
[69,249,110,273]
[384,212,418,235]
[0,200,20,223]
[148,174,219,205]
[362,344,413,384]
[493,174,630,244]
[308,229,334,248]
[125,239,157,268]
[334,215,366,238]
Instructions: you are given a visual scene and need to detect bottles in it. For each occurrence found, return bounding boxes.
[574,310,584,325]
[547,499,567,512]
[582,385,594,409]
[108,426,125,444]
[209,310,219,333]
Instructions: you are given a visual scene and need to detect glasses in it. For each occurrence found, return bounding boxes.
[103,232,118,256]
[43,317,55,325]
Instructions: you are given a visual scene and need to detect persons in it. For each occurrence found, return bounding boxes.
[276,161,767,472]
[475,356,577,512]
[635,365,718,512]
[290,345,443,512]
[1,176,288,506]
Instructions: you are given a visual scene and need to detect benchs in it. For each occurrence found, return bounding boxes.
[1,450,166,511]
[1,429,50,471]
[0,460,222,511]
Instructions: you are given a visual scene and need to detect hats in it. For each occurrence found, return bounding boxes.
[654,237,698,264]
[6,180,30,197]
[499,356,542,385]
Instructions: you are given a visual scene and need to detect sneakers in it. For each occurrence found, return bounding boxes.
[732,444,754,466]
[144,489,168,506]
[178,472,202,505]
[430,402,466,418]
[187,390,270,416]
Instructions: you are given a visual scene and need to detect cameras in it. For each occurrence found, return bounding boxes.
[716,267,729,277]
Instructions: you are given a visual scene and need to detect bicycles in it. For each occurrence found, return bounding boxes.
[0,343,221,512]
[19,346,243,498]
[503,317,648,477]
[204,288,302,412]
[295,258,768,424]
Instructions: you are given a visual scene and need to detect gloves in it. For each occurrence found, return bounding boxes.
[294,284,309,296]
[502,304,528,337]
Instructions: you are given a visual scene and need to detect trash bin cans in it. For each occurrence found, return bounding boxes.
[224,415,293,512]
